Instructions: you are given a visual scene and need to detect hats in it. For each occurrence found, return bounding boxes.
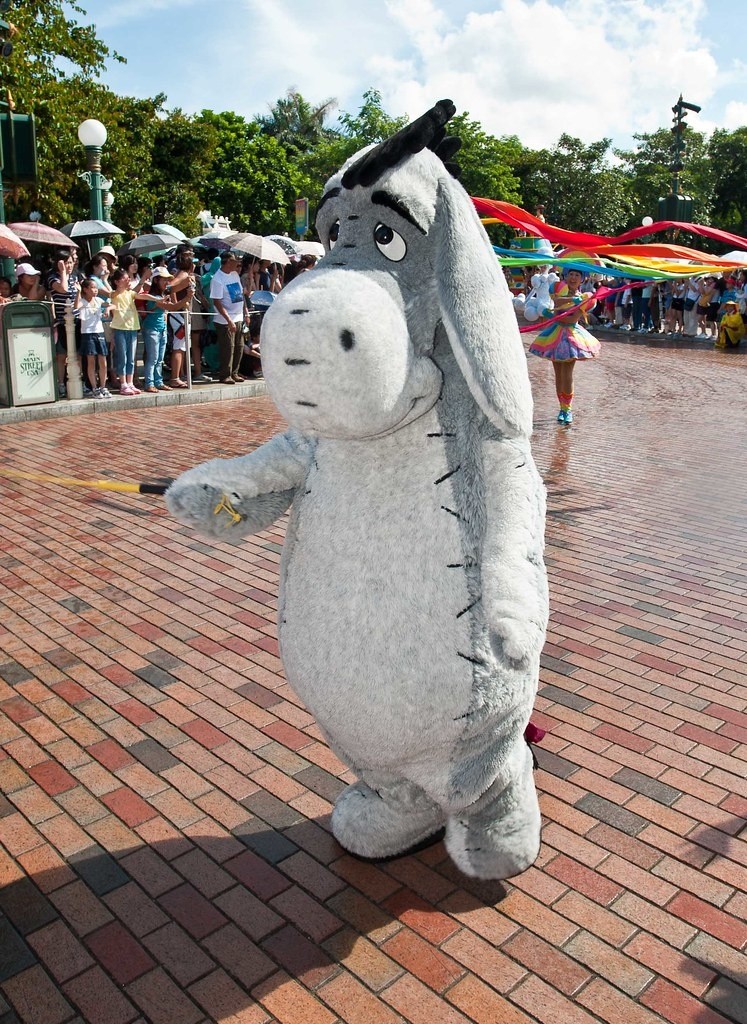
[149,266,174,282]
[97,245,117,263]
[16,263,41,277]
[722,300,739,311]
[725,278,735,285]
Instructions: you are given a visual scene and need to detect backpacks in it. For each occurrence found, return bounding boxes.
[134,291,164,320]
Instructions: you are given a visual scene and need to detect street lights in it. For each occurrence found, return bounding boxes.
[77,118,114,261]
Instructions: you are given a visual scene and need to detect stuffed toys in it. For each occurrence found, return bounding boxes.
[168,99,551,881]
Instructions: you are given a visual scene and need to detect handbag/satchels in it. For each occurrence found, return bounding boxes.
[684,298,695,311]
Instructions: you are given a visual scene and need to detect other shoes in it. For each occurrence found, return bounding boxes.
[170,380,188,387]
[220,374,245,385]
[253,369,262,378]
[194,374,213,382]
[145,387,159,393]
[159,385,173,390]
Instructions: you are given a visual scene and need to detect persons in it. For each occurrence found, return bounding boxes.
[530,205,546,235]
[578,269,747,347]
[511,249,565,328]
[0,243,316,398]
[529,248,602,423]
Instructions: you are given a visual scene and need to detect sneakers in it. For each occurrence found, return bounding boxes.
[82,382,92,396]
[58,383,67,397]
[120,384,141,395]
[91,388,113,398]
[557,410,573,423]
[661,328,717,340]
[583,321,659,334]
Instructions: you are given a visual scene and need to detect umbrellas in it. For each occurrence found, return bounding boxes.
[0,218,326,273]
[651,250,747,267]
